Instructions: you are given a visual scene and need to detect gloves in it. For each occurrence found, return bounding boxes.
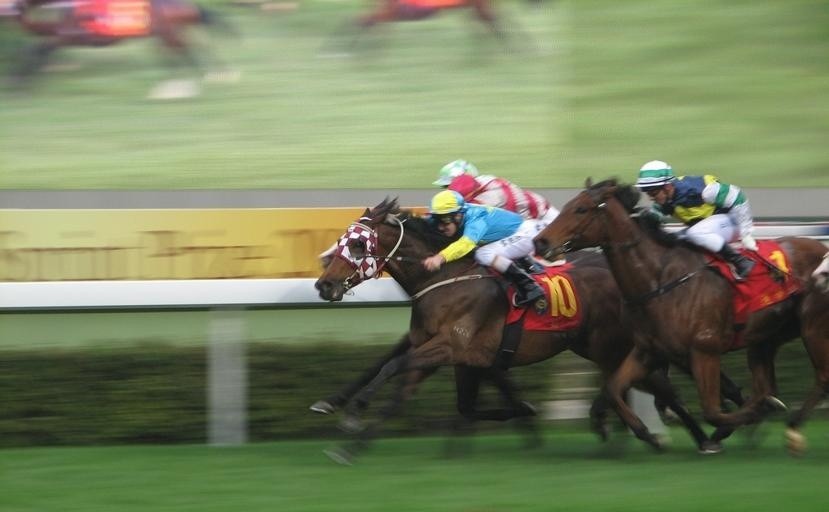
[743,236,760,251]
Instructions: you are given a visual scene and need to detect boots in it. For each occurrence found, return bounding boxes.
[520,251,548,275]
[720,242,755,278]
[505,263,547,317]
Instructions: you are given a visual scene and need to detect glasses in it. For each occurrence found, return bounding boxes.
[641,185,666,196]
[429,212,457,224]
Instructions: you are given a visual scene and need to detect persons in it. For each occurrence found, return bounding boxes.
[632,161,758,279]
[434,159,559,225]
[423,190,547,309]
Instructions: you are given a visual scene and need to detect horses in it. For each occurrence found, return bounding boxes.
[535,177,829,455]
[362,0,503,42]
[308,193,635,439]
[0,0,211,79]
[315,193,688,426]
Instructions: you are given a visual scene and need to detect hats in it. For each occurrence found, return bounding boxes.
[448,175,479,199]
[633,158,677,188]
[424,191,469,216]
[434,157,478,185]
